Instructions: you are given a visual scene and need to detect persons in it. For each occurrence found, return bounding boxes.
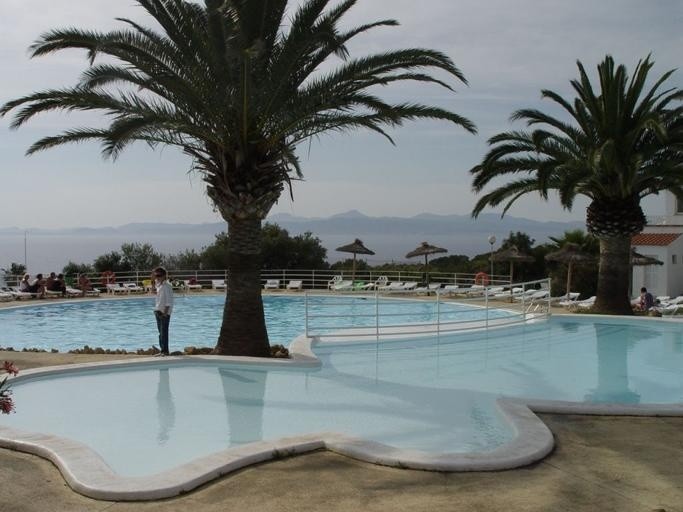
[20,273,90,299]
[151,274,197,286]
[635,287,653,312]
[150,267,174,357]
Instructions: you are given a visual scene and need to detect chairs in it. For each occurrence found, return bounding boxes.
[328,279,595,311]
[0,279,303,301]
[631,296,683,317]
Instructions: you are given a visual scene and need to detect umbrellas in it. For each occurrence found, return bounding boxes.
[489,244,536,295]
[405,242,448,289]
[544,242,599,302]
[336,238,375,286]
[627,246,664,298]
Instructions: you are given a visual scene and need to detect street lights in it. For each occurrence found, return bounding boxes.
[486,233,495,286]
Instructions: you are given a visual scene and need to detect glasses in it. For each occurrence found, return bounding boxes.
[155,274,163,278]
